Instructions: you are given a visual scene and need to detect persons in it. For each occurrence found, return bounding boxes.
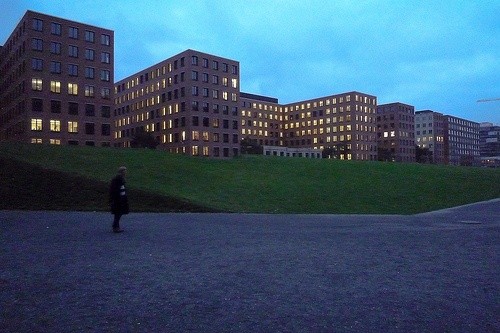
[108,166,131,234]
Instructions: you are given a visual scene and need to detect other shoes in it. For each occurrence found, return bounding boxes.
[113,229,123,233]
